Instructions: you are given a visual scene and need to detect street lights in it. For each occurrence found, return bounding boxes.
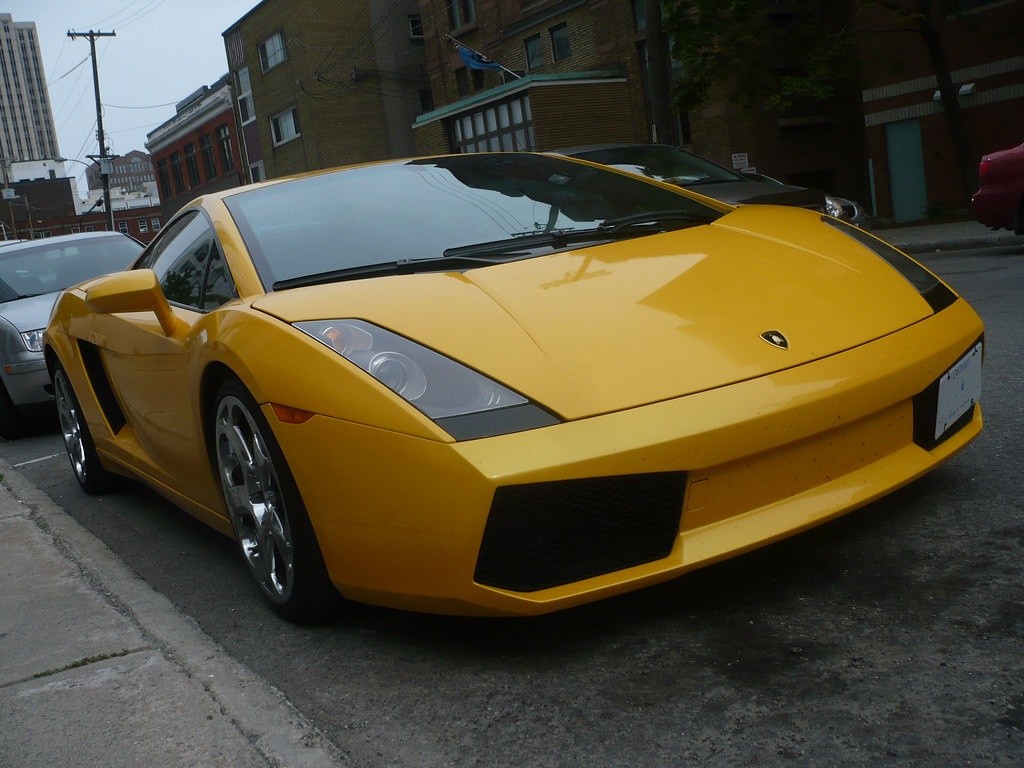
[53,156,115,231]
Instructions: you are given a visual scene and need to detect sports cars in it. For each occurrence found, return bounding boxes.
[41,150,986,626]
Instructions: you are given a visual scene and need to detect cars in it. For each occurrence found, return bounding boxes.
[755,174,875,235]
[546,141,828,221]
[969,142,1023,237]
[0,238,62,286]
[0,231,149,441]
